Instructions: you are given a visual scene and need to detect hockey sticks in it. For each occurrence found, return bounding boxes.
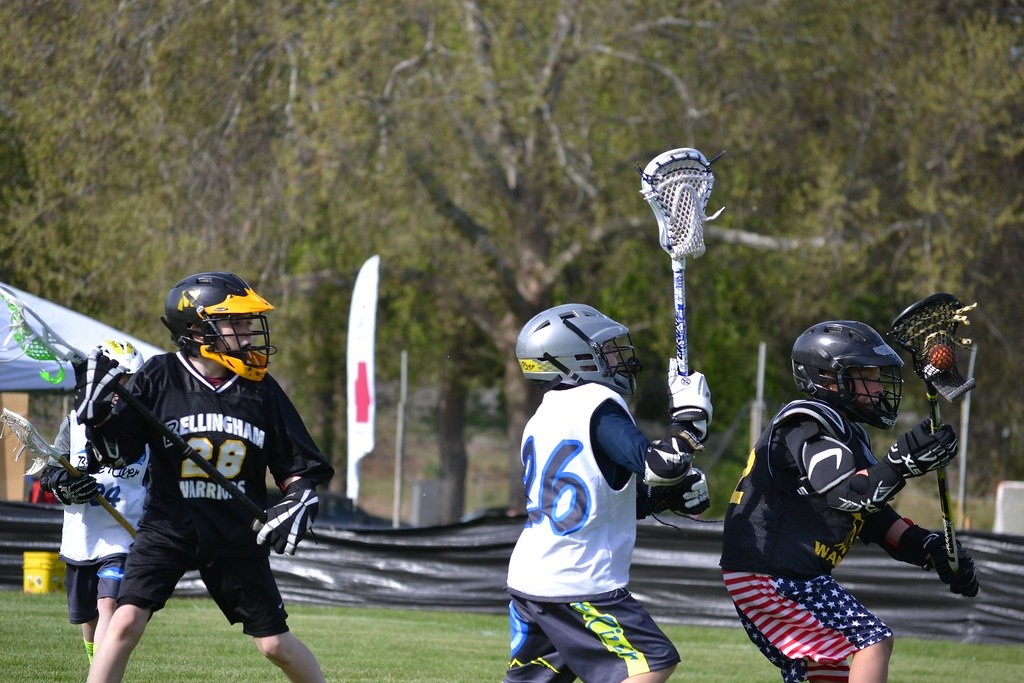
[0,406,137,540]
[885,291,978,577]
[8,301,268,525]
[634,146,731,478]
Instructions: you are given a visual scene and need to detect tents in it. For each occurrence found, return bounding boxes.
[0,284,167,393]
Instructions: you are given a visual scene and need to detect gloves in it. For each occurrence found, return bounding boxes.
[665,357,713,445]
[252,478,319,557]
[71,345,131,427]
[646,467,711,515]
[40,466,100,507]
[913,530,980,598]
[883,416,960,480]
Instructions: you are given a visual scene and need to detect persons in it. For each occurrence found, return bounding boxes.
[72,272,336,683]
[720,321,980,683]
[39,339,149,665]
[502,304,713,683]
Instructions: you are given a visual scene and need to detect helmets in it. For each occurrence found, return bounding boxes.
[791,319,905,430]
[160,272,277,382]
[91,340,145,374]
[514,302,644,397]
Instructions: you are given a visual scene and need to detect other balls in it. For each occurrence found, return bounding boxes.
[928,343,953,371]
